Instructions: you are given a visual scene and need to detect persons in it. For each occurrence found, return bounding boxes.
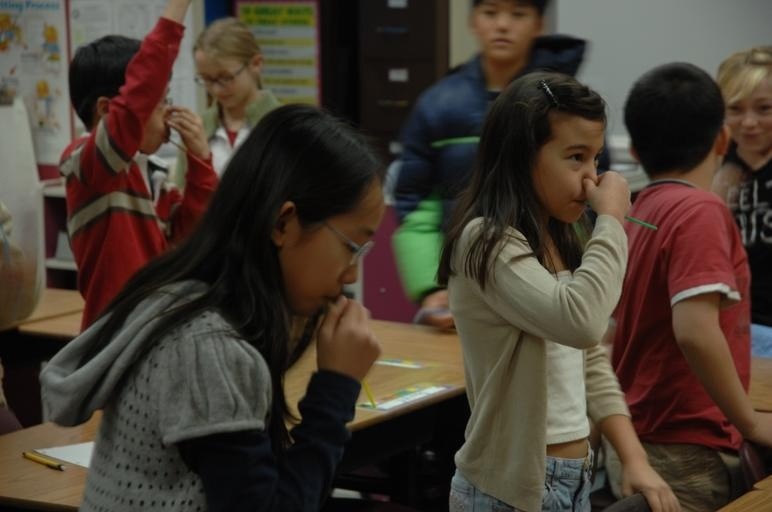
[386,2,610,338]
[167,15,290,197]
[60,2,222,342]
[0,196,40,435]
[583,59,771,510]
[700,44,772,331]
[432,66,686,510]
[37,99,385,509]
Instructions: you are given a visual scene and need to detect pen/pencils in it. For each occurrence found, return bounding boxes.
[22,451,66,471]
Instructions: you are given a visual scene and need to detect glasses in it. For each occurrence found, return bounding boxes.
[324,218,378,266]
[193,72,243,86]
